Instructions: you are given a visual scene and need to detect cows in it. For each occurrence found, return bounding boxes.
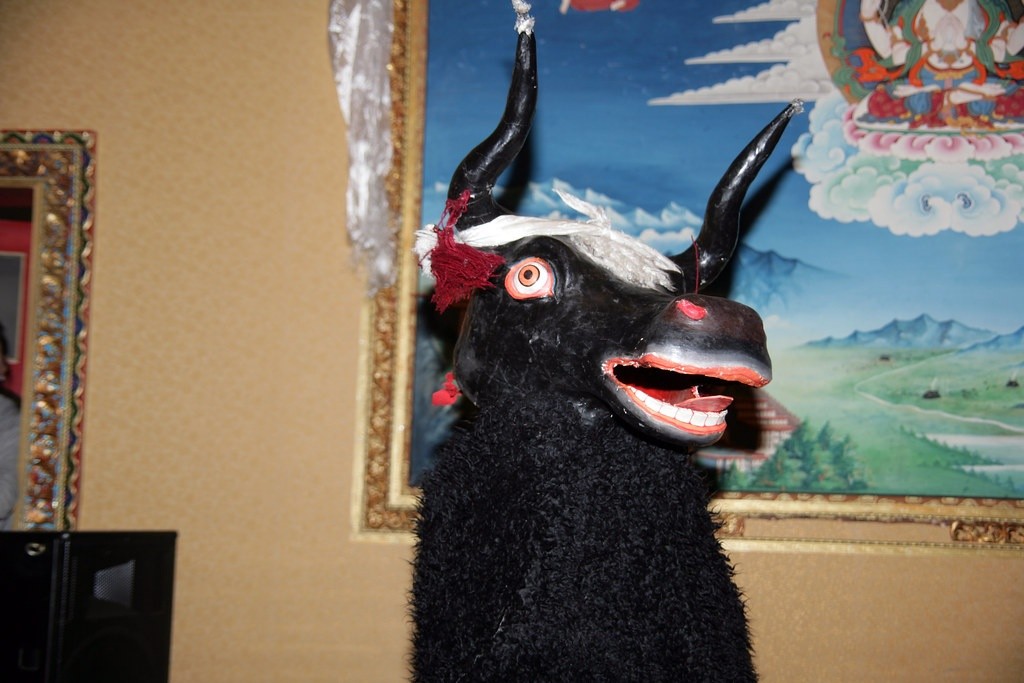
[401,13,802,681]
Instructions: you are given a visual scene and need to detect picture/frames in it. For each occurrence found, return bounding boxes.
[353,1,1024,547]
[0,128,96,533]
